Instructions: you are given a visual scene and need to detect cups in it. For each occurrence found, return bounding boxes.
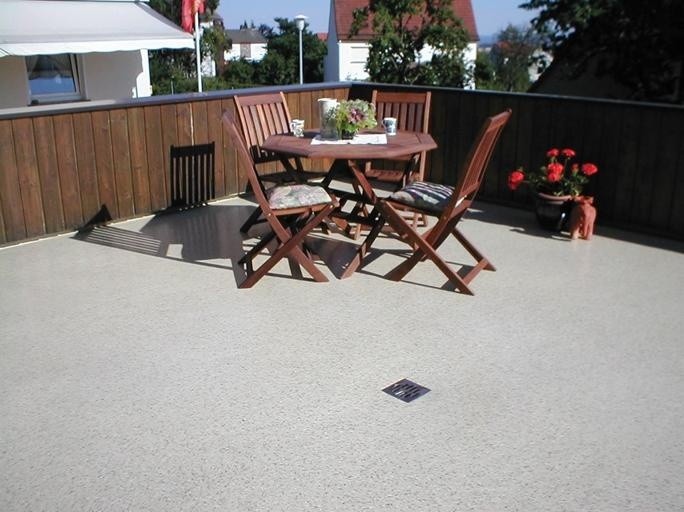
[290,119,304,137]
[381,117,397,136]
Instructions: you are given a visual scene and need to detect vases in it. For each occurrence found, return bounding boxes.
[341,129,353,140]
[535,191,571,231]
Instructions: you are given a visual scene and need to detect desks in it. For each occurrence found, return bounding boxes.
[260,127,439,279]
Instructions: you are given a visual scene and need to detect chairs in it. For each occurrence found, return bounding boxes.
[233,91,348,235]
[222,110,342,288]
[352,89,432,240]
[376,107,512,296]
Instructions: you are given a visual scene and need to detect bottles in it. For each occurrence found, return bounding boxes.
[317,98,340,137]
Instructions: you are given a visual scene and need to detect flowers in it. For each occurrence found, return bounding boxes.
[323,99,378,139]
[507,147,599,196]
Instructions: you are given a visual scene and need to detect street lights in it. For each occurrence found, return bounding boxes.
[294,15,308,84]
